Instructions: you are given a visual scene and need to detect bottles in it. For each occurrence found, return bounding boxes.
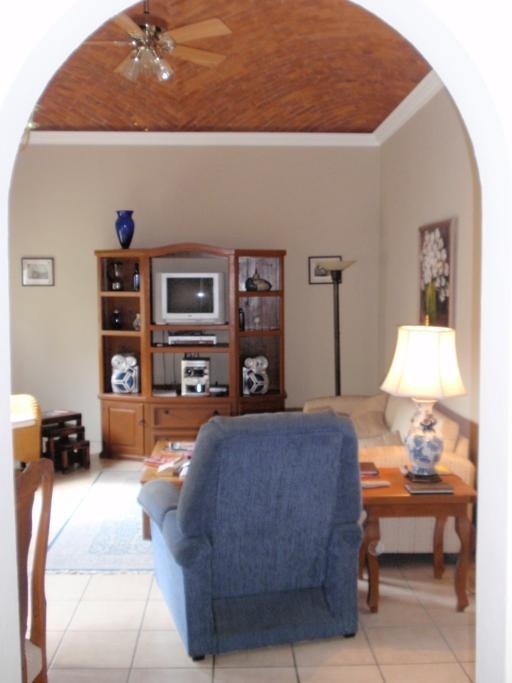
[239,308,244,331]
[132,313,141,330]
[112,264,123,291]
[112,310,122,330]
[132,263,139,292]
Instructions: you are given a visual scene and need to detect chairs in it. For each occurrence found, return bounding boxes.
[136,411,359,661]
[14,457,56,683]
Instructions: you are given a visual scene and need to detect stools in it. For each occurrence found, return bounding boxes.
[43,424,85,461]
[45,440,90,474]
[42,408,82,425]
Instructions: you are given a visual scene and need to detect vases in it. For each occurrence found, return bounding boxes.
[115,210,135,248]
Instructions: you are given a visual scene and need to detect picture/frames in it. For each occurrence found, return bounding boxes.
[308,256,341,284]
[418,217,457,326]
[21,257,55,286]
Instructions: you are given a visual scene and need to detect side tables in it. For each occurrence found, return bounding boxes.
[359,467,477,614]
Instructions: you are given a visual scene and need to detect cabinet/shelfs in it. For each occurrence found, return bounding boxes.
[94,241,286,461]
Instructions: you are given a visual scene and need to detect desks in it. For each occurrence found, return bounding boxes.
[12,393,43,463]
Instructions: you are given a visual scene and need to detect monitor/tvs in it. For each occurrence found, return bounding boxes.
[155,272,225,325]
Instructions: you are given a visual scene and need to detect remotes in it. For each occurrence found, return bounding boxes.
[361,480,390,488]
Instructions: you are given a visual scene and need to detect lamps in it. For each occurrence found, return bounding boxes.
[377,325,468,480]
[315,259,357,397]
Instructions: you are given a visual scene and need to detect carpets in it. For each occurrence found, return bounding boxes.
[41,471,152,575]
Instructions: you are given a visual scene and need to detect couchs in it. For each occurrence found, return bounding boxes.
[303,396,477,565]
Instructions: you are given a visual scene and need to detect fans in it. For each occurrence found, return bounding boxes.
[82,0,232,83]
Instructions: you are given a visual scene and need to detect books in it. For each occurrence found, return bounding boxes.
[404,482,453,494]
[359,463,378,477]
